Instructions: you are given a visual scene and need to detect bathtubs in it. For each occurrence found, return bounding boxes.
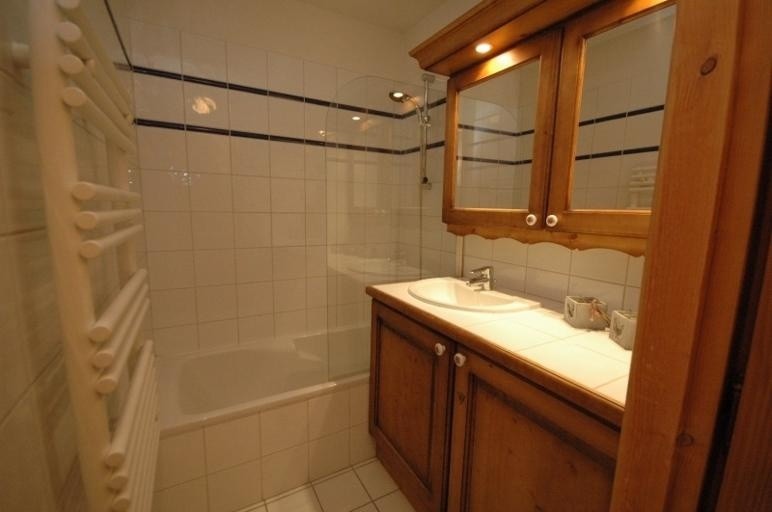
[155,320,379,439]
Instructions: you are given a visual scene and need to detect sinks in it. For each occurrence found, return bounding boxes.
[408,275,541,312]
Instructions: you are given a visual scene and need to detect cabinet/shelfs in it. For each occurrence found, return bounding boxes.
[365,295,621,512]
[441,0,678,257]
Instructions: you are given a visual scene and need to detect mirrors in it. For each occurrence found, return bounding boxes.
[570,0,677,210]
[453,55,541,210]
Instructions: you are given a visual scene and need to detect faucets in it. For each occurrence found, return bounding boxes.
[466,265,494,291]
